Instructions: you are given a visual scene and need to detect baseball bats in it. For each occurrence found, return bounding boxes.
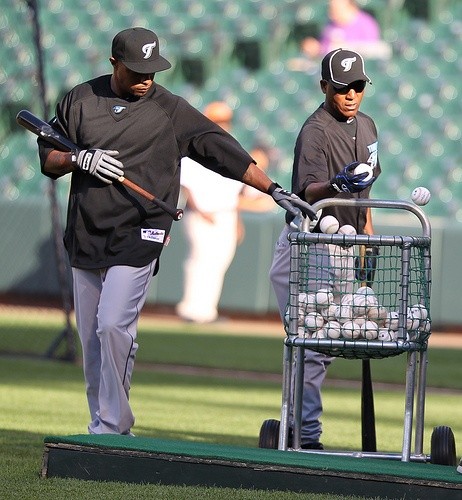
[15,109,184,222]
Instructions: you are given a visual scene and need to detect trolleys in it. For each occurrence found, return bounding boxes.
[257,198,457,465]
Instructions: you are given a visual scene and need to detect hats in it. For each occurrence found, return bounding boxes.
[111,26,173,74]
[321,49,372,89]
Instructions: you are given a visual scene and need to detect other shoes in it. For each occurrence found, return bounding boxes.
[300,442,324,449]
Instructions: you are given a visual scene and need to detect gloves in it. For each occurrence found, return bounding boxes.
[325,161,374,193]
[356,244,380,287]
[270,182,319,222]
[70,148,124,185]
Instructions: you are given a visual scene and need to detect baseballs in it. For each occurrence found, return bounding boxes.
[411,186,431,206]
[283,286,431,356]
[319,215,358,236]
[352,163,374,181]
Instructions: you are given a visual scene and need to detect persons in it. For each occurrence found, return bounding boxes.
[268,48,381,450]
[176,101,244,322]
[237,149,275,212]
[36,27,317,436]
[300,0,383,59]
